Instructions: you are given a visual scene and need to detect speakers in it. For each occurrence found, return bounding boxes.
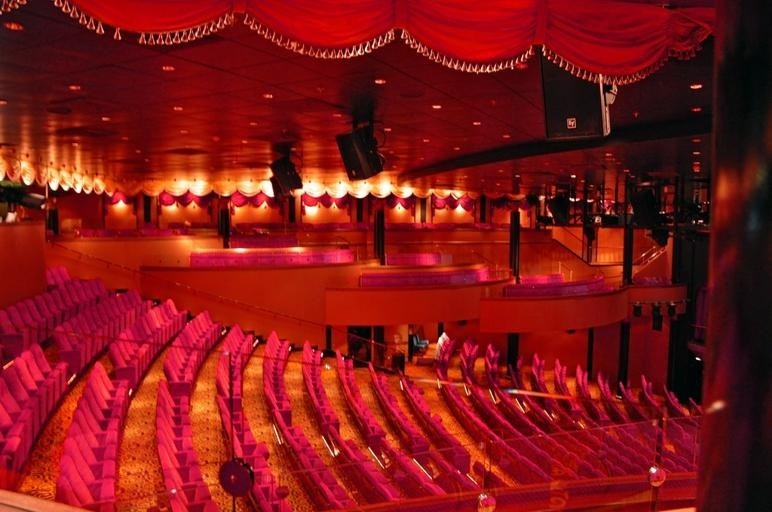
[269,163,304,191]
[540,51,613,143]
[336,130,383,181]
